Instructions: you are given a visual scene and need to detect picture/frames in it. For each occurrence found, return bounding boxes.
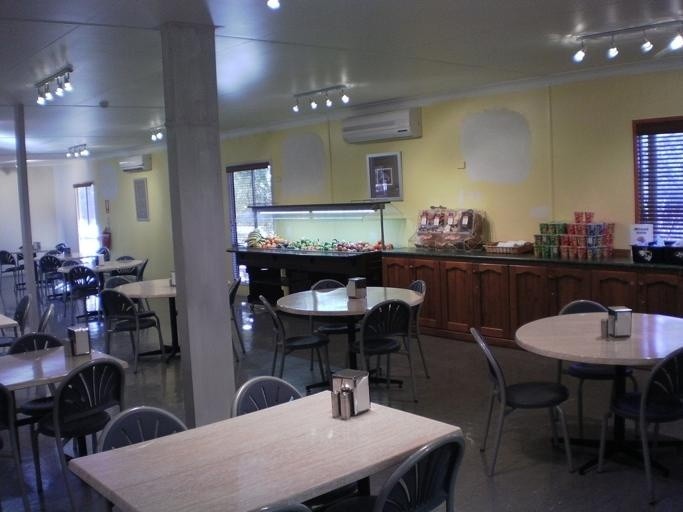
[366,151,404,202]
[133,178,150,220]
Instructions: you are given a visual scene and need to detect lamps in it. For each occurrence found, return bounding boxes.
[66,142,89,157]
[34,67,73,106]
[291,85,350,111]
[573,16,683,62]
[149,125,166,142]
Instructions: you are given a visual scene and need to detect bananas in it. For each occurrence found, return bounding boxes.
[245,231,262,246]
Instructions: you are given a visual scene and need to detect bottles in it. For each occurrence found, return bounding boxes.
[338,386,352,421]
[330,391,340,418]
[62,338,71,357]
[600,319,608,337]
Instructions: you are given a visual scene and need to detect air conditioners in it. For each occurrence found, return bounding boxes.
[118,153,152,174]
[341,106,421,144]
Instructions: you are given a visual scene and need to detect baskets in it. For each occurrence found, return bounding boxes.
[482,240,533,254]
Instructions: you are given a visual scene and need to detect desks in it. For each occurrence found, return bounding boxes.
[515,311,683,477]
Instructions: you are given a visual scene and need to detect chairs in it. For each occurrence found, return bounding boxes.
[597,348,683,506]
[556,300,637,452]
[470,325,574,478]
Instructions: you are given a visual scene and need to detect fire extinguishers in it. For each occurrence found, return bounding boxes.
[102,227,111,249]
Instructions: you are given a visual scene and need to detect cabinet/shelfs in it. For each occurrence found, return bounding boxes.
[440,261,510,348]
[592,269,683,319]
[509,264,592,350]
[226,199,408,311]
